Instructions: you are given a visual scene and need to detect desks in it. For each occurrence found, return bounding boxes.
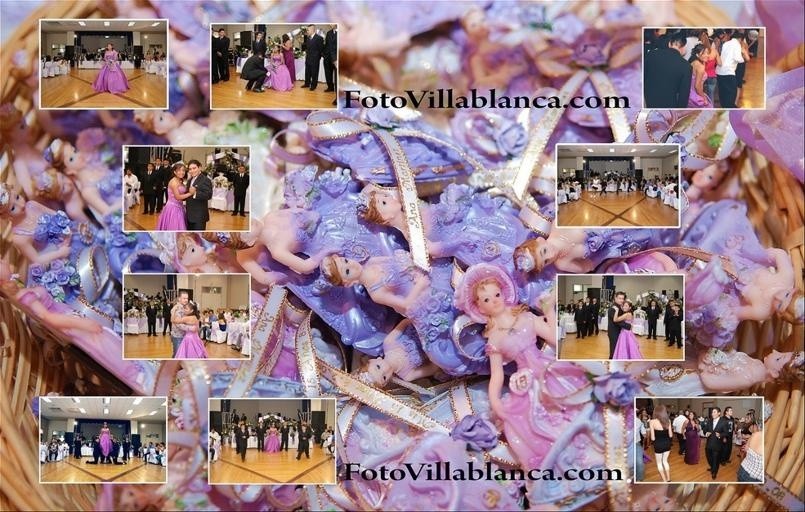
[78,59,134,70]
[124,315,172,335]
[598,316,684,338]
[163,187,249,212]
[588,179,637,192]
[236,57,327,84]
[81,443,134,458]
[229,430,315,449]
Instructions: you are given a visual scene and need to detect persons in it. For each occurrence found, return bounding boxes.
[1,2,805,511]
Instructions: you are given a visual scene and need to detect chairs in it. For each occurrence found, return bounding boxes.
[558,313,577,340]
[210,434,221,464]
[646,185,678,209]
[39,443,69,461]
[558,188,580,205]
[319,431,335,460]
[123,182,141,212]
[41,60,71,78]
[141,59,166,79]
[138,448,166,465]
[199,321,249,356]
